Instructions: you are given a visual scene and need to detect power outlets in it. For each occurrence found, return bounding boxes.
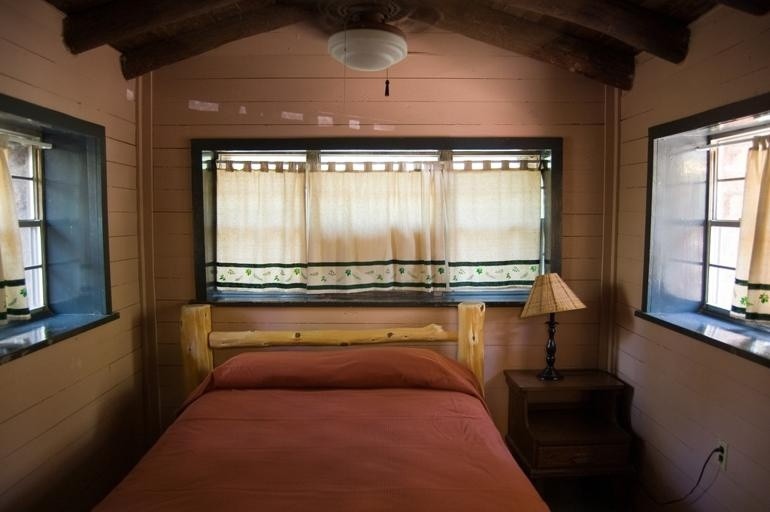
[715,441,728,470]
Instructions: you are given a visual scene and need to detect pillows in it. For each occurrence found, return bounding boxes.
[213,345,475,396]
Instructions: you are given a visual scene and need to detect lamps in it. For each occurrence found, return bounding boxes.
[312,0,410,72]
[520,272,589,381]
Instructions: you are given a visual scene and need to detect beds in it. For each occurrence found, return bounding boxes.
[93,303,552,512]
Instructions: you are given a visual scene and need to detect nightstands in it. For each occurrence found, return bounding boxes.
[503,369,636,511]
